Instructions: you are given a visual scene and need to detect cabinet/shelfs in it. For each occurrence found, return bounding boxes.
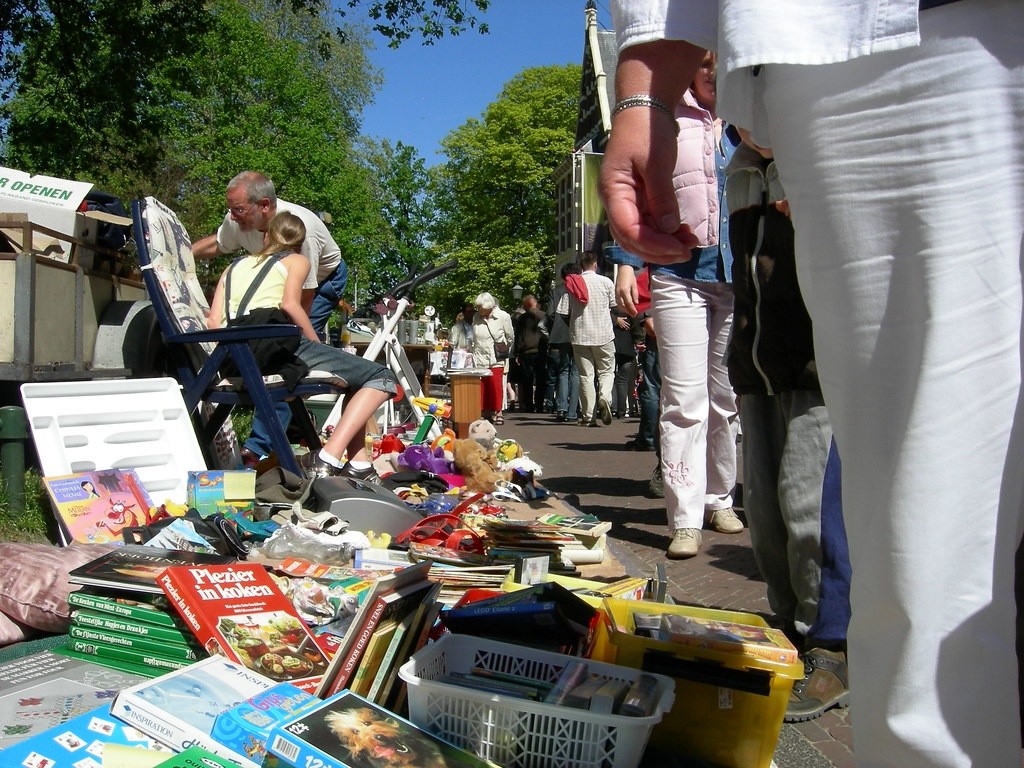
[450,375,481,424]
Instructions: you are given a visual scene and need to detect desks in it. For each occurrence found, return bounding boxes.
[353,343,454,398]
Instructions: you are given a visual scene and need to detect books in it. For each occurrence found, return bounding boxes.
[65,514,614,768]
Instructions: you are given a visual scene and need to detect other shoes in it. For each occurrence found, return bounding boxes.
[554,416,565,424]
[577,420,598,427]
[567,418,578,424]
[649,464,666,498]
[598,398,612,425]
[305,452,343,480]
[340,460,384,491]
[625,435,655,451]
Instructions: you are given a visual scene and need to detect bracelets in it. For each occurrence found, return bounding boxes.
[610,94,680,137]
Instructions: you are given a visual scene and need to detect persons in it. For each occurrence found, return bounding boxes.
[784,436,852,721]
[610,51,742,558]
[451,291,546,426]
[207,211,398,483]
[596,0,1024,768]
[546,250,662,451]
[193,171,348,462]
[723,127,834,638]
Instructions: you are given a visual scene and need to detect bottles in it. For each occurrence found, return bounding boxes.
[440,339,448,347]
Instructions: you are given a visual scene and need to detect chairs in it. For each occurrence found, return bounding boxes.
[132,196,349,479]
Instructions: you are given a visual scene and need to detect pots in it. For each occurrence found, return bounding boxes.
[253,635,314,680]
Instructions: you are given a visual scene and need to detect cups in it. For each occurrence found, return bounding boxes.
[365,436,373,462]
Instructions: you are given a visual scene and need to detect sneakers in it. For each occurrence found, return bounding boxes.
[705,508,744,533]
[668,527,702,558]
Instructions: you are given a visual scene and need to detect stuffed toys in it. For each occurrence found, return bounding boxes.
[398,420,543,493]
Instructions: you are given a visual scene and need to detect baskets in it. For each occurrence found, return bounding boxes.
[397,634,676,768]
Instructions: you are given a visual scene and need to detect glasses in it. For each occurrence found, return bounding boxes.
[226,198,261,216]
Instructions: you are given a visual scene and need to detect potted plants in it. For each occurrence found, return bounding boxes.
[328,309,345,342]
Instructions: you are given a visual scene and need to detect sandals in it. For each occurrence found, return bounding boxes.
[496,416,504,426]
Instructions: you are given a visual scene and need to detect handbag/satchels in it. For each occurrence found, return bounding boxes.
[495,342,509,361]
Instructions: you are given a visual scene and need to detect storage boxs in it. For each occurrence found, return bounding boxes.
[397,634,676,768]
[0,166,134,271]
[599,597,806,768]
